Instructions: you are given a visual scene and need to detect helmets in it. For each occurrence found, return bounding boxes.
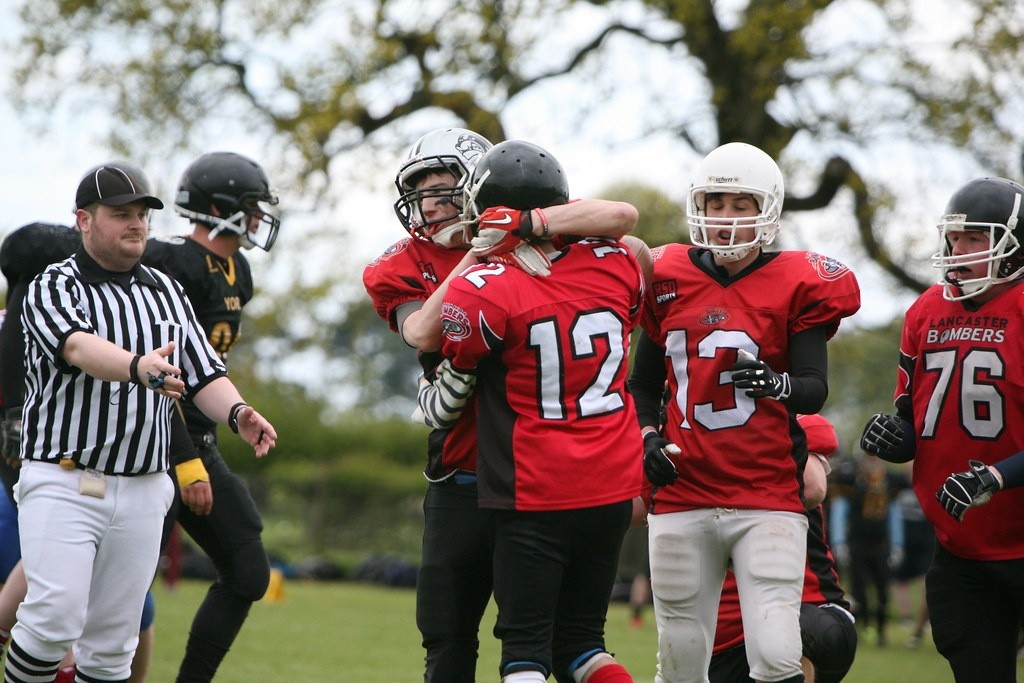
[173,152,282,254]
[392,127,495,249]
[683,142,785,265]
[460,139,572,241]
[930,176,1023,301]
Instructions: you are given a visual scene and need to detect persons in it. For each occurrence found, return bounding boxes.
[360,127,1023,683]
[0,152,278,683]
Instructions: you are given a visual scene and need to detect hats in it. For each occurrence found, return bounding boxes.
[75,163,165,213]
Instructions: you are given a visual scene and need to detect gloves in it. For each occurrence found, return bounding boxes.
[642,430,682,487]
[860,411,903,458]
[475,228,553,281]
[731,348,793,399]
[469,205,541,259]
[931,458,1001,523]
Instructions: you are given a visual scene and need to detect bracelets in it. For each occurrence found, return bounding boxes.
[228,403,250,436]
[130,354,140,385]
[535,207,548,236]
